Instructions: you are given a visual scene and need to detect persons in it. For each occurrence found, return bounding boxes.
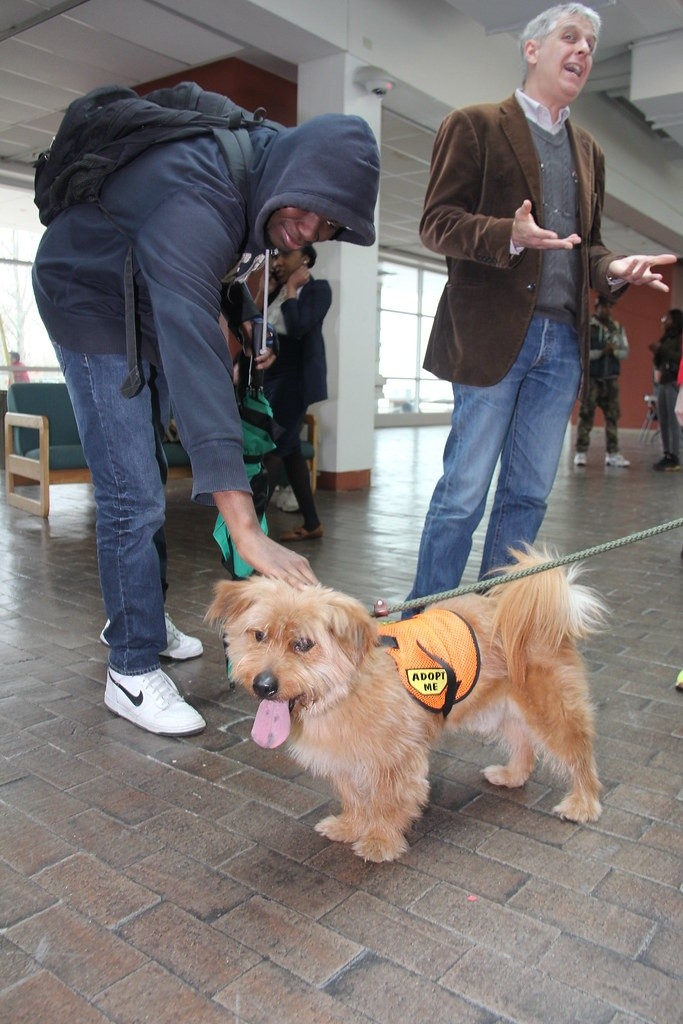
[229,238,334,542]
[399,2,679,625]
[648,308,683,472]
[7,351,29,389]
[574,296,630,468]
[28,111,382,736]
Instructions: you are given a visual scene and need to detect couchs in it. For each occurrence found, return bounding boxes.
[5,381,318,518]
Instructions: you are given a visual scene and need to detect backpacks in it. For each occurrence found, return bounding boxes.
[34,82,287,236]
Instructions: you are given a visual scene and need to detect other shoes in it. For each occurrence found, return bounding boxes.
[653,455,683,472]
[280,523,324,542]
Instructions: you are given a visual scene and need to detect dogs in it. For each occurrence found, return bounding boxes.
[203,539,617,864]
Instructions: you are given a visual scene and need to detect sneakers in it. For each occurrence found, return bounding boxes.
[104,666,206,737]
[99,613,203,658]
[574,452,586,466]
[605,453,630,467]
